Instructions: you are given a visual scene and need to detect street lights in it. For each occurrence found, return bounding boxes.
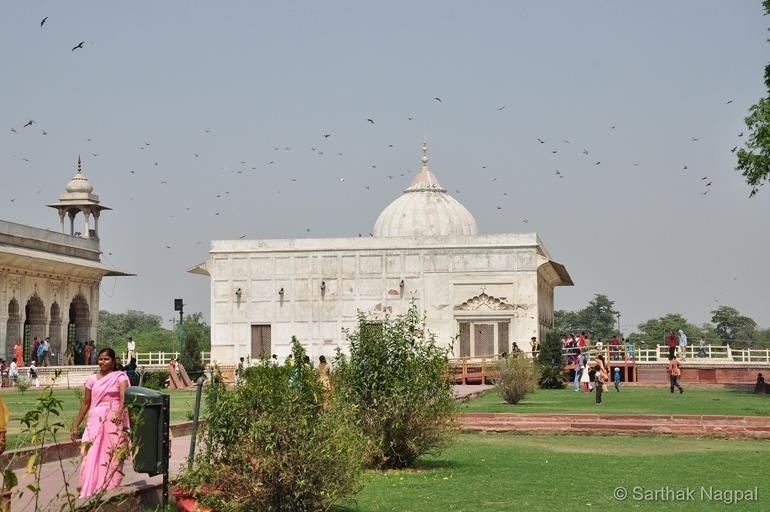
[174,298,184,355]
[169,316,175,334]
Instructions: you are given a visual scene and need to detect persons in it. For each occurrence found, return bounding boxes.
[666,330,687,394]
[497,331,633,404]
[166,358,180,381]
[70,347,131,499]
[696,336,707,358]
[0,335,96,387]
[237,354,331,390]
[0,397,9,457]
[115,337,139,386]
[753,373,764,394]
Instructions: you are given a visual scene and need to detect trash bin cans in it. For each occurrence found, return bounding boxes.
[124,386,170,512]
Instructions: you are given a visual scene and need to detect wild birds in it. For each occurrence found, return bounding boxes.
[215,96,528,238]
[72,41,85,50]
[537,126,616,179]
[1,120,211,204]
[40,16,48,26]
[683,99,745,196]
